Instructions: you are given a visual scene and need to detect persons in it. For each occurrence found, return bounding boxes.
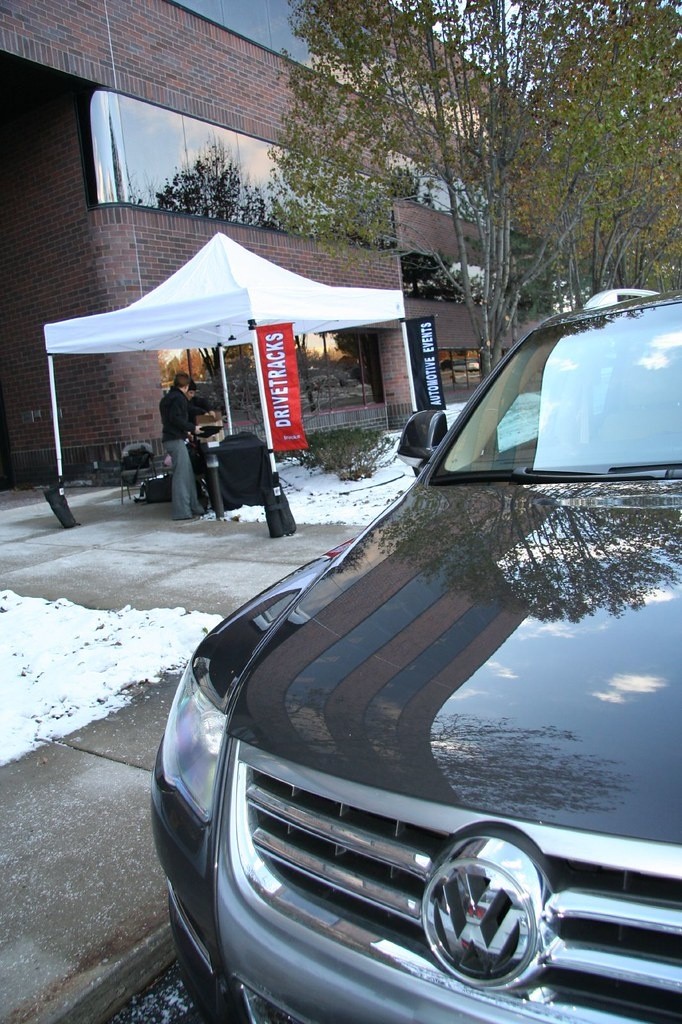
[159,373,216,520]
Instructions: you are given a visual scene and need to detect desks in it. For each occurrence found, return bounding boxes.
[201,432,275,512]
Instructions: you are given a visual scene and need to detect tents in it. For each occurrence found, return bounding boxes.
[42,230,420,540]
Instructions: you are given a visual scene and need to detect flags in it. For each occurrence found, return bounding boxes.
[404,314,446,412]
[254,323,310,453]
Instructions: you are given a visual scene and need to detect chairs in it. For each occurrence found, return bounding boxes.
[119,443,156,505]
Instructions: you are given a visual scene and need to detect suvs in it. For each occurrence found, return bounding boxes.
[148,290,682,1024]
